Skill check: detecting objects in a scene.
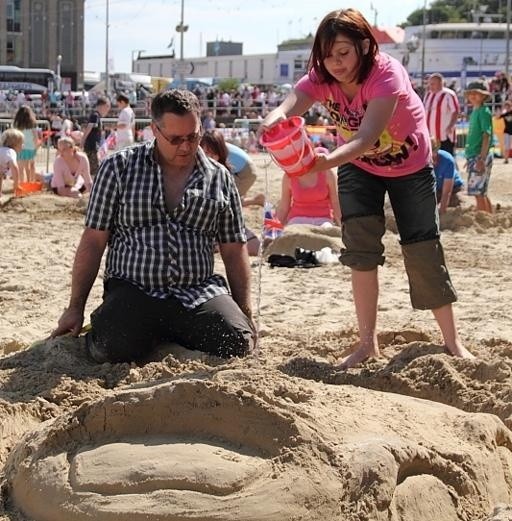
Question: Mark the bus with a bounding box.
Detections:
[0,66,61,93]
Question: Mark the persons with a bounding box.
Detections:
[53,88,256,363]
[258,8,478,370]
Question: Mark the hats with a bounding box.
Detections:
[461,79,493,103]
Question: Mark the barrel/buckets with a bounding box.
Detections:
[259,115,318,177]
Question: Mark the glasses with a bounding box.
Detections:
[151,119,205,147]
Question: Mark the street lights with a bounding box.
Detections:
[367,3,378,25]
[420,0,429,90]
[57,54,63,92]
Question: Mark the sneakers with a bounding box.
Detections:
[83,331,110,366]
[266,253,296,268]
[295,246,325,266]
